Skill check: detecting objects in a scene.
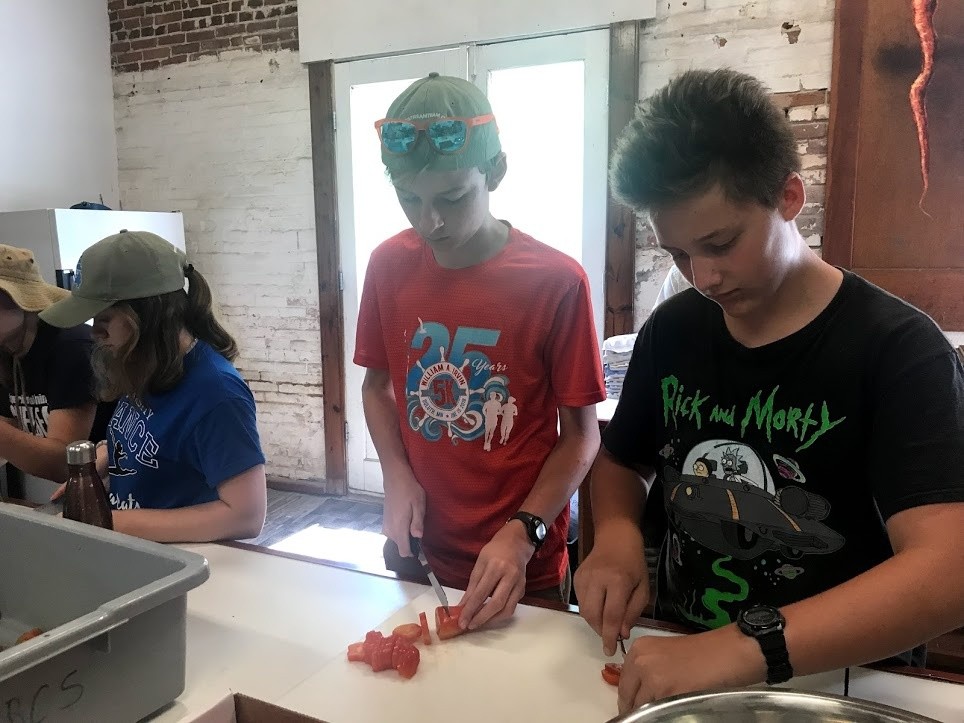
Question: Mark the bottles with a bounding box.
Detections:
[62,440,114,532]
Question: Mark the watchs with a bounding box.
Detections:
[506,511,548,551]
[737,603,793,685]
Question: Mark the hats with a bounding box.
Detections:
[38,229,187,328]
[382,72,502,171]
[0,243,71,312]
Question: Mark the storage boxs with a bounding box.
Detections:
[0,500,209,722]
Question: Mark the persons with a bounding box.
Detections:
[352,73,607,632]
[49,231,267,543]
[570,66,964,717]
[0,244,121,485]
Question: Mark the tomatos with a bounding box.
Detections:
[347,604,486,679]
[601,661,624,685]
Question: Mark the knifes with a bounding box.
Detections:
[409,533,448,613]
[617,634,628,660]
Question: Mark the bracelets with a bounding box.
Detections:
[96,440,107,449]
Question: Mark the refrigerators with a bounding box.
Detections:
[0,207,190,326]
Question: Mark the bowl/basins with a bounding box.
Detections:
[605,687,945,723]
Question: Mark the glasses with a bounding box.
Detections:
[374,114,497,156]
